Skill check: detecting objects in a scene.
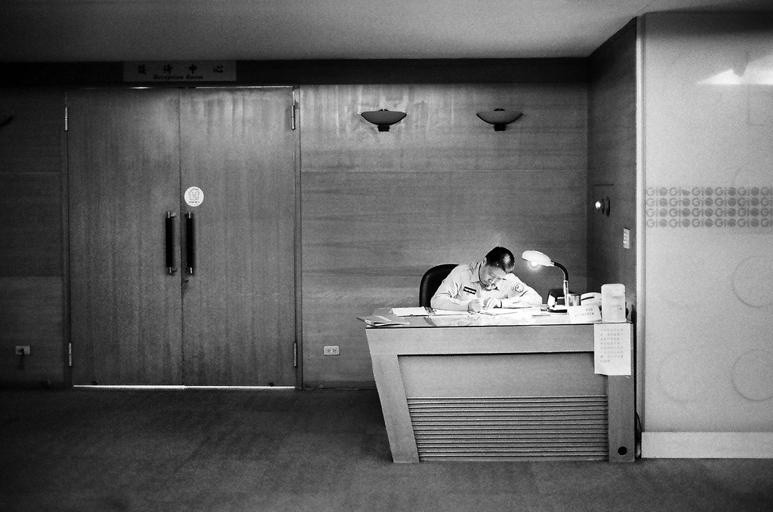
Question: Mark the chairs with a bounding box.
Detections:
[419,264,459,306]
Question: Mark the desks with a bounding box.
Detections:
[365,306,635,463]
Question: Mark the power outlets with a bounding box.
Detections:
[323,346,339,356]
[15,345,31,356]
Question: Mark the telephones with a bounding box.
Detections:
[580,292,603,307]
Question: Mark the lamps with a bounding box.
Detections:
[592,198,612,215]
[361,109,406,132]
[477,108,522,131]
[521,250,571,317]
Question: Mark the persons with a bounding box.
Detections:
[430,246,543,312]
